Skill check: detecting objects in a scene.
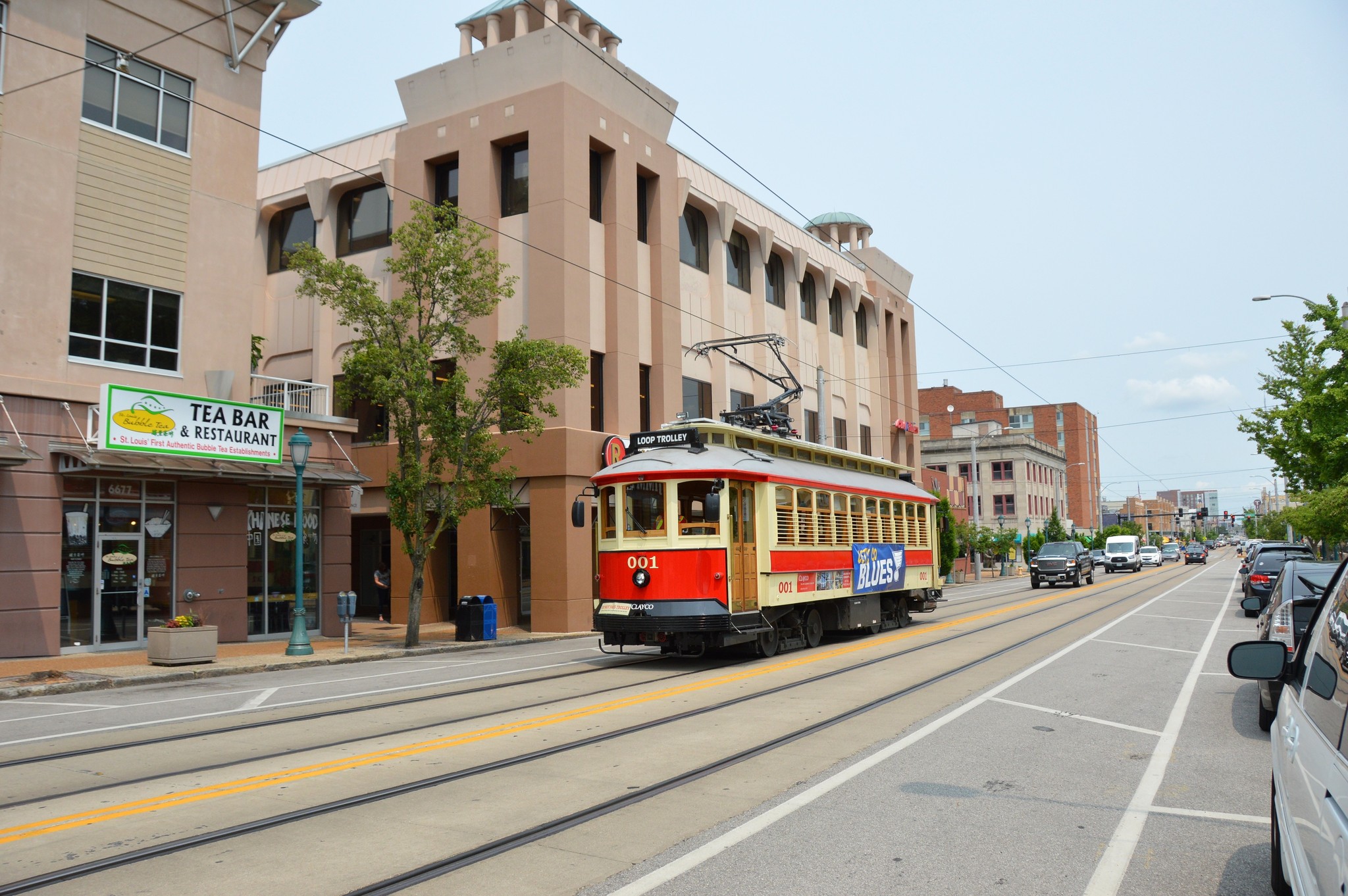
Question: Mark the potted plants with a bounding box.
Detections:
[1005,564,1015,576]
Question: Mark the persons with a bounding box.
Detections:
[374,562,390,621]
[653,494,687,529]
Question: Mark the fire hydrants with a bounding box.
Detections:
[1017,564,1024,575]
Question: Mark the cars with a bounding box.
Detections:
[1238,560,1344,729]
[1226,556,1348,896]
[1327,608,1348,666]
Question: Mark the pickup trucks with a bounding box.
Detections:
[1029,534,1319,614]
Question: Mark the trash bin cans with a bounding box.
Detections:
[455,596,484,641]
[477,595,496,640]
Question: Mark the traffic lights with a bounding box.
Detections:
[1173,531,1184,538]
[1197,512,1203,520]
[1224,511,1251,528]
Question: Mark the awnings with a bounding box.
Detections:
[0,444,44,465]
[50,446,374,487]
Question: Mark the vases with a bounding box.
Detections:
[148,626,218,663]
[951,572,964,583]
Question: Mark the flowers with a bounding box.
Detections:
[955,569,963,573]
[161,611,205,628]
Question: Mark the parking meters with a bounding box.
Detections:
[337,591,356,653]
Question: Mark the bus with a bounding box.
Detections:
[571,334,949,660]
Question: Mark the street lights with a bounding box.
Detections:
[1099,482,1122,533]
[1145,501,1162,546]
[1250,475,1279,515]
[1025,517,1032,572]
[997,514,1007,576]
[1089,525,1094,551]
[1043,519,1050,544]
[1127,493,1147,522]
[1250,452,1293,543]
[972,427,1014,578]
[1249,496,1265,514]
[1160,505,1175,546]
[283,425,315,655]
[1072,524,1076,541]
[1249,488,1273,518]
[1056,462,1086,525]
